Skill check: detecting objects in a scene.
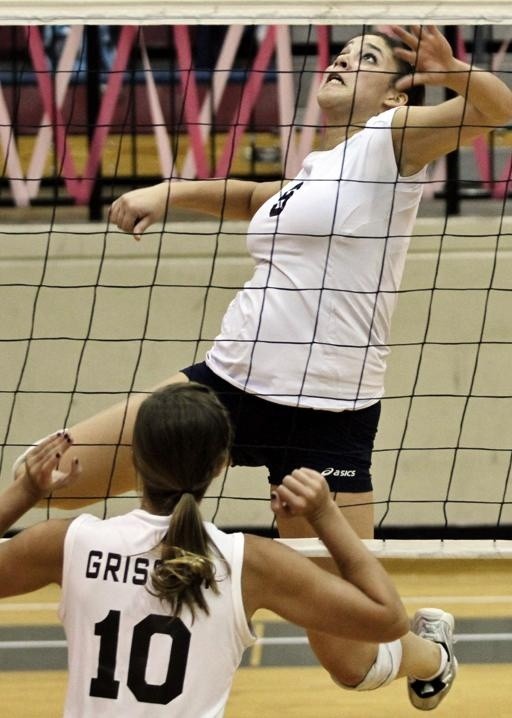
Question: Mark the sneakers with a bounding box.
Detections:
[409,609,455,712]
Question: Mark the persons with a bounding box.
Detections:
[2,379,414,717]
[14,22,509,712]
